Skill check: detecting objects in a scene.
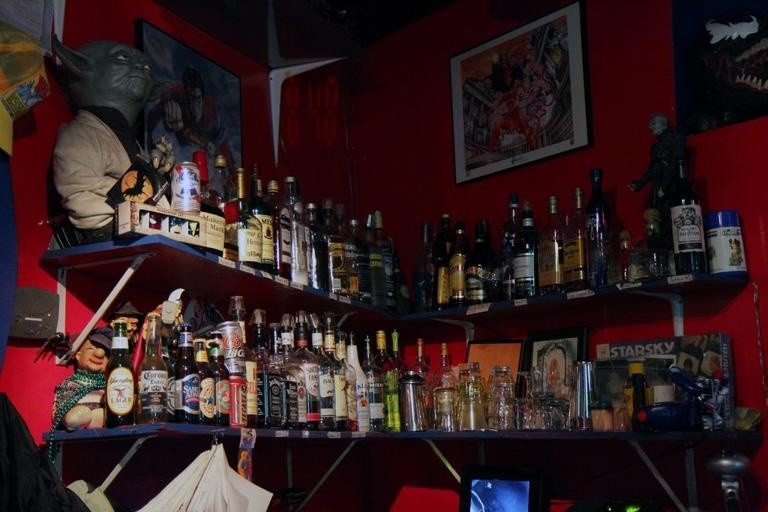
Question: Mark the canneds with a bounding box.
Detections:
[170,161,203,214]
[228,377,249,427]
[215,320,247,375]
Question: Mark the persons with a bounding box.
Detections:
[674,208,702,228]
[52,286,186,430]
[626,117,691,229]
[148,66,239,172]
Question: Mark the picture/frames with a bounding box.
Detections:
[447,1,594,185]
[514,327,589,428]
[134,17,244,188]
[464,337,526,399]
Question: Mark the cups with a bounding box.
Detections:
[149,210,161,229]
[169,215,182,234]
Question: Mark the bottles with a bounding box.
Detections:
[137,179,170,217]
[191,144,407,309]
[666,158,709,276]
[410,164,665,314]
[103,293,657,430]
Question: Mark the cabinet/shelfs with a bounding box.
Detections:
[39,233,397,443]
[396,270,760,443]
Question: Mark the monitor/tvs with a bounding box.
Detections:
[459,463,545,512]
